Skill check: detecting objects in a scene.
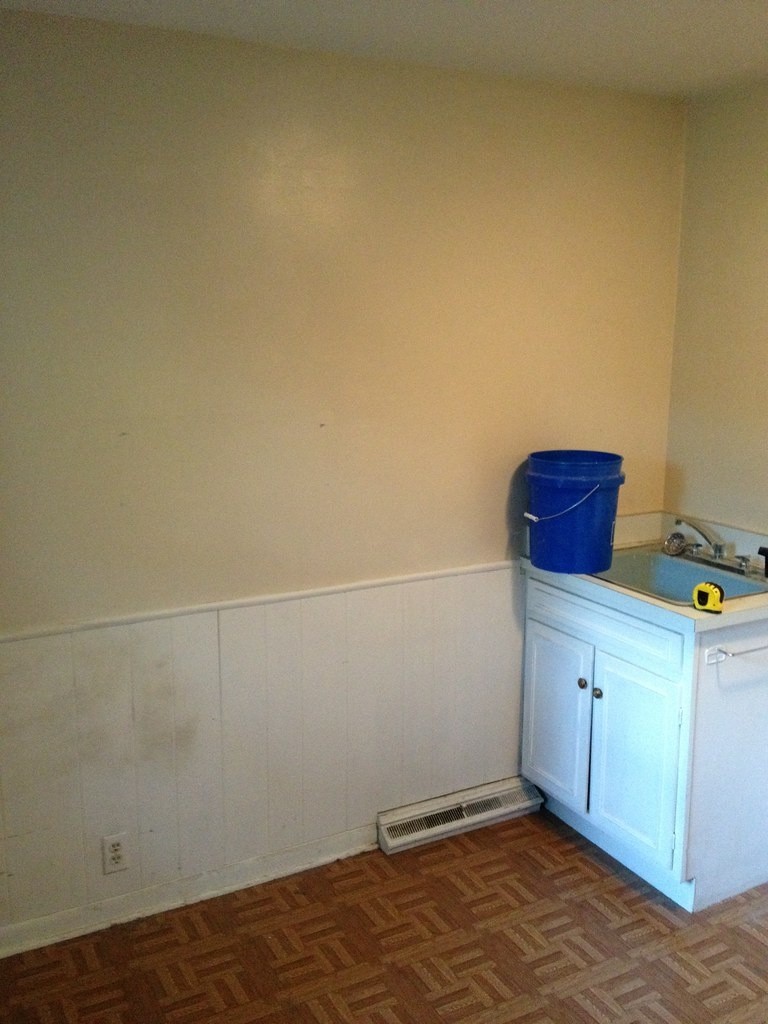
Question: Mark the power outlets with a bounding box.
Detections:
[101,832,129,875]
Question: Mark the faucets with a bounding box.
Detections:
[673,518,726,557]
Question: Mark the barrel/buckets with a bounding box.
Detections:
[524,451,625,574]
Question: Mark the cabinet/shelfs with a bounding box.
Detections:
[521,577,768,913]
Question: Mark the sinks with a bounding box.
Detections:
[581,540,768,605]
